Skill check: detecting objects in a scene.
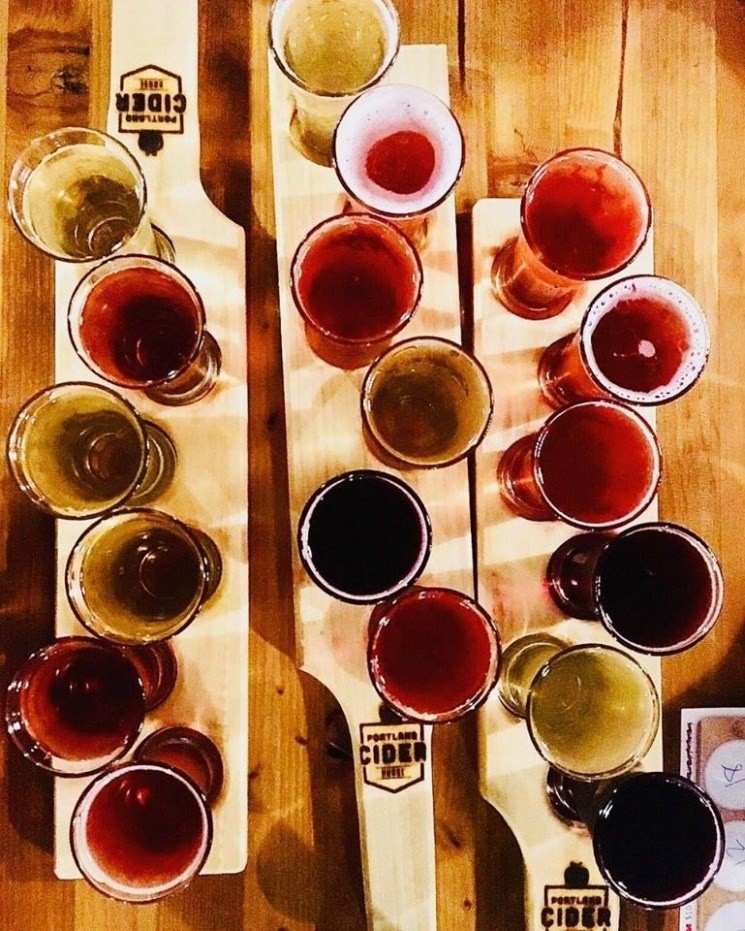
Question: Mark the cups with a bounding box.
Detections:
[296,469,432,605]
[65,508,210,646]
[289,212,425,370]
[267,0,400,153]
[509,398,664,532]
[66,253,216,400]
[365,586,503,725]
[360,336,494,471]
[3,381,153,520]
[545,522,725,656]
[5,635,160,777]
[68,744,214,905]
[331,83,466,236]
[496,632,660,782]
[7,126,159,273]
[512,147,652,307]
[546,762,726,910]
[544,274,711,405]
